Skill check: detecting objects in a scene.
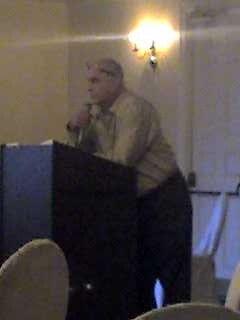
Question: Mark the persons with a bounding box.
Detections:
[61,59,192,313]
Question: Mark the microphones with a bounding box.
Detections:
[76,102,92,146]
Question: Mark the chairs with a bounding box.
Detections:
[1,239,71,319]
[135,303,239,319]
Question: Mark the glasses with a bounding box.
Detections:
[85,61,113,77]
